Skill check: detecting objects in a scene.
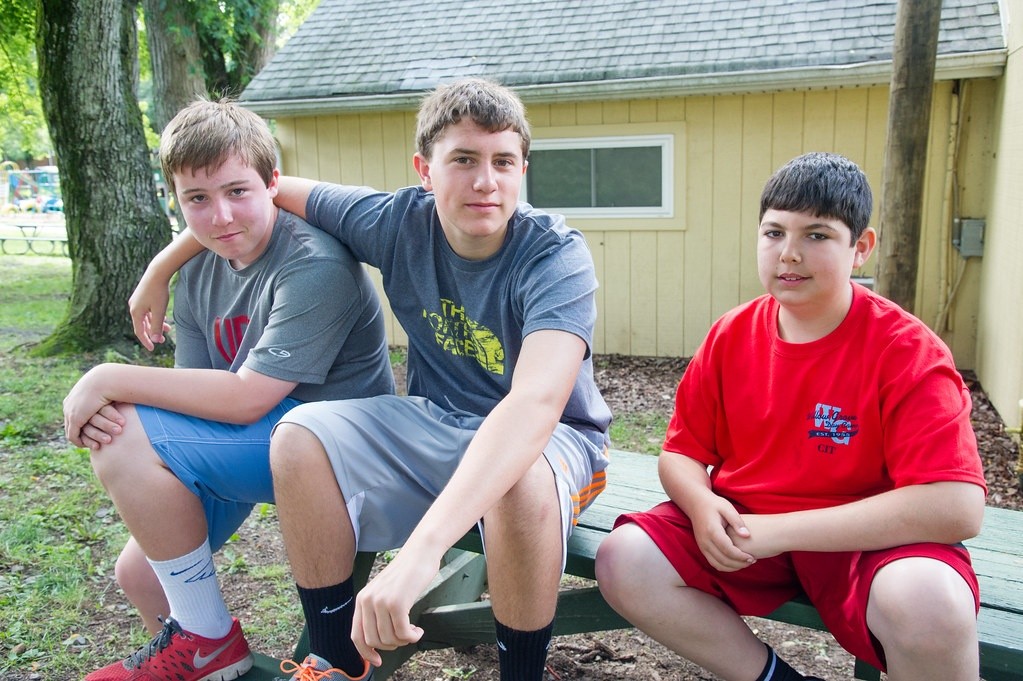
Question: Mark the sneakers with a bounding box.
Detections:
[81,615,253,681]
[279,652,375,681]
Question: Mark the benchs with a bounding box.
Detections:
[238,450,1022,681]
[0,238,69,257]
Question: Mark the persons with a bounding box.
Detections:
[129,78,613,681]
[594,153,988,680]
[63,103,396,680]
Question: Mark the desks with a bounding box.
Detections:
[17,225,39,255]
